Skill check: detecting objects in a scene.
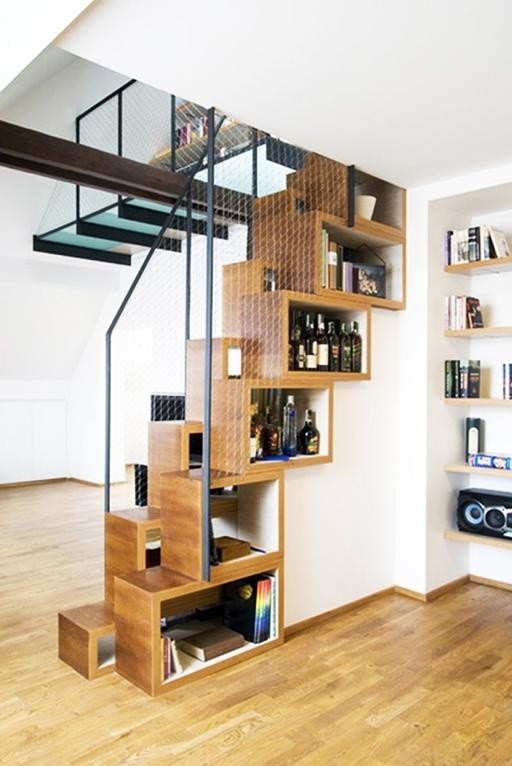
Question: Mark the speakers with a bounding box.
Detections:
[455,483,512,540]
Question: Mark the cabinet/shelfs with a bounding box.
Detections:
[113,164,407,697]
[441,254,509,548]
[58,153,318,682]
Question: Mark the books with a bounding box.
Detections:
[446,225,509,265]
[447,294,485,329]
[177,628,245,662]
[446,359,482,398]
[162,635,183,681]
[219,574,275,642]
[322,226,352,292]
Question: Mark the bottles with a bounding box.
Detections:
[316,314,329,371]
[338,323,352,372]
[299,409,319,455]
[282,395,297,457]
[254,402,260,461]
[348,321,362,372]
[291,309,305,371]
[303,314,319,371]
[260,406,279,457]
[326,322,338,371]
[250,405,256,463]
